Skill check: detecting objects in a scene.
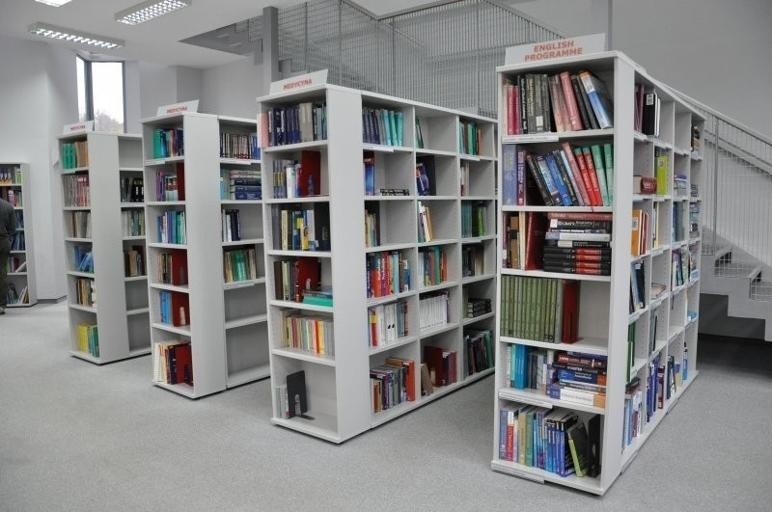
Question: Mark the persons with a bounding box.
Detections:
[0,197,19,316]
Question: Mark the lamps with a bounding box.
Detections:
[35,0,74,10]
[117,0,192,27]
[27,24,126,53]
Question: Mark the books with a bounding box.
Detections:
[60,140,101,359]
[621,76,702,457]
[0,164,30,305]
[219,126,263,284]
[457,121,495,380]
[502,143,614,207]
[150,124,195,387]
[256,99,336,421]
[416,116,459,395]
[503,71,612,135]
[119,172,148,278]
[502,212,613,478]
[360,104,416,416]
[623,76,650,455]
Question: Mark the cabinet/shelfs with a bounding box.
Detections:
[253,82,497,446]
[139,111,269,400]
[494,51,708,494]
[2,162,38,308]
[56,130,152,365]
